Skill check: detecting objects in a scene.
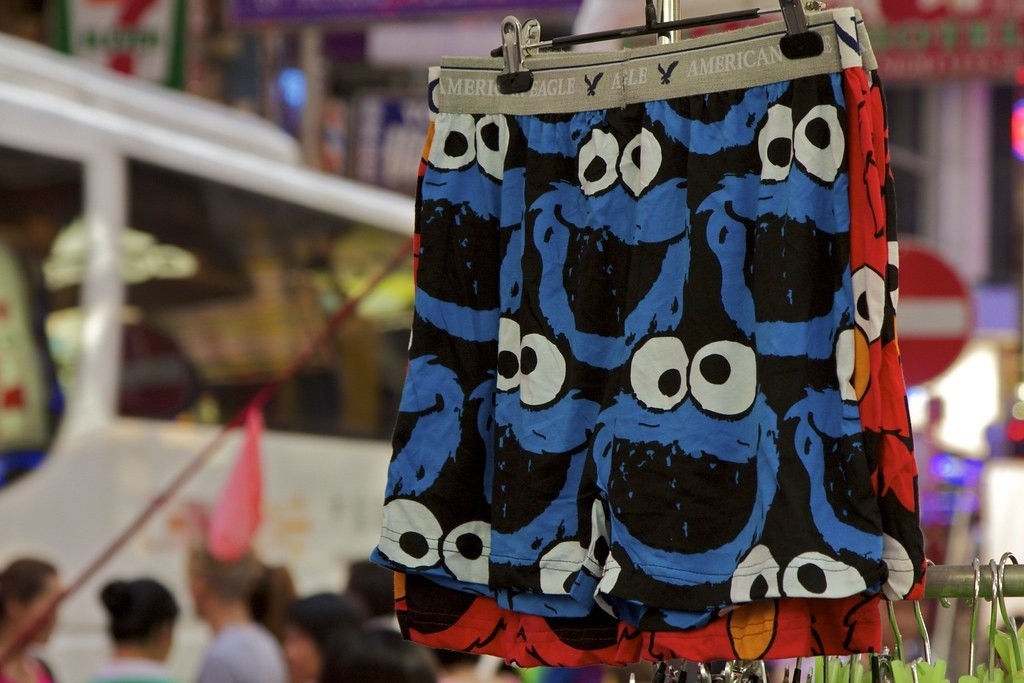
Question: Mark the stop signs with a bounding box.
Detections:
[893,246,979,394]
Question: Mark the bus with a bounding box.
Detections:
[0,35,427,672]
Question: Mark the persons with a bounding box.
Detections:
[185,536,482,683]
[87,579,178,683]
[911,396,945,564]
[0,559,62,683]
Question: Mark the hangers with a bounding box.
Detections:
[488,0,826,94]
[647,554,1024,683]
